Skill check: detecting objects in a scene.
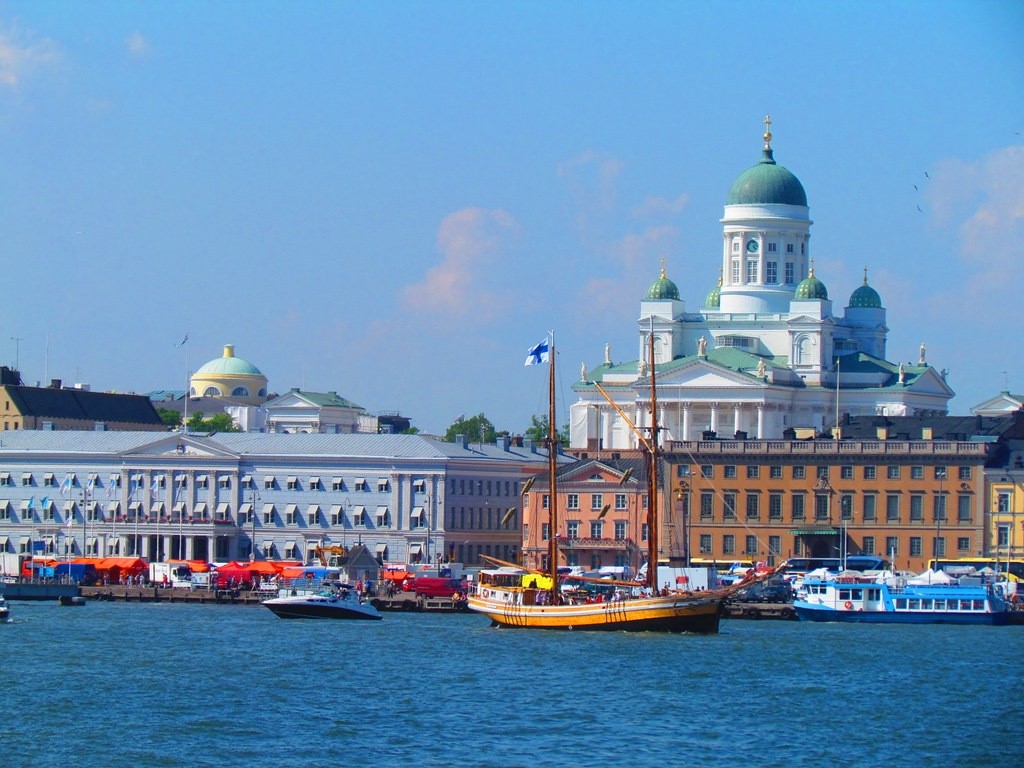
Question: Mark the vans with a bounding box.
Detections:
[415,577,465,600]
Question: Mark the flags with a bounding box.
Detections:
[128,479,137,500]
[175,483,181,503]
[524,337,549,366]
[66,516,72,527]
[28,497,34,508]
[41,496,48,509]
[106,478,115,497]
[86,476,93,492]
[60,473,71,494]
[149,481,158,500]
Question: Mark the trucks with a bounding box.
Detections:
[152,565,193,587]
[1,553,39,584]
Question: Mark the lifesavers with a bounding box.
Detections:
[403,599,413,610]
[845,601,852,609]
[370,598,381,609]
[781,607,792,618]
[720,608,727,618]
[483,589,489,598]
[747,608,759,619]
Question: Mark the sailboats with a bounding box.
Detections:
[465,315,792,634]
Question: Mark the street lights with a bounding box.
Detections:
[79,486,430,564]
[684,471,696,568]
[936,470,945,558]
[993,495,1006,567]
[838,491,847,571]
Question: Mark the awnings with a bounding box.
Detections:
[0,472,424,554]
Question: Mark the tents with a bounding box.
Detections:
[22,558,302,588]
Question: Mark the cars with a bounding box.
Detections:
[0,596,11,619]
[561,572,617,595]
[739,583,789,603]
[402,579,415,592]
[460,580,475,588]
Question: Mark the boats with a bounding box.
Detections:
[260,578,384,623]
[794,568,1012,625]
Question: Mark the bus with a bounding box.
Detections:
[639,557,756,576]
[315,547,349,568]
[783,557,892,572]
[928,558,1024,590]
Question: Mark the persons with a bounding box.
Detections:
[899,363,904,382]
[162,573,167,588]
[920,343,925,362]
[536,585,667,606]
[251,576,258,591]
[581,335,766,380]
[356,579,468,601]
[941,369,948,379]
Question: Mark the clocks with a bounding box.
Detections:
[746,239,760,252]
